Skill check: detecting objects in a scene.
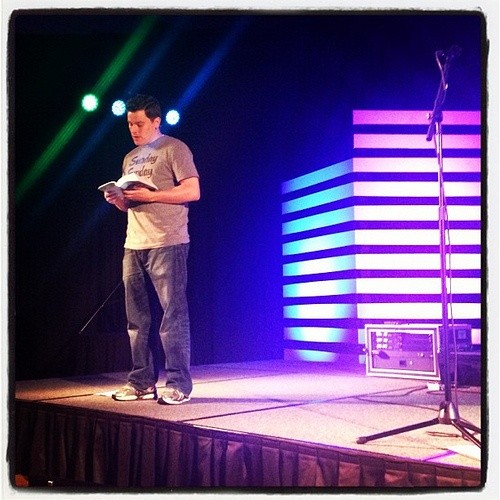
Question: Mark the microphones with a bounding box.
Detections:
[436,49,451,90]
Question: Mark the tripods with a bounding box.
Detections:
[357,82,483,452]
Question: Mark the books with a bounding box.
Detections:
[99,173,160,195]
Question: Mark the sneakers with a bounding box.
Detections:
[158,387,191,404]
[112,382,157,402]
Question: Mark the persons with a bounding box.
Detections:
[103,93,203,405]
